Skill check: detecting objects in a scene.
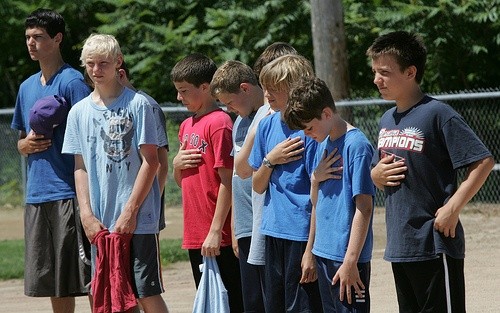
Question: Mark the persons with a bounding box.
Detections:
[367,29,496,312]
[171,40,373,312]
[11,6,170,312]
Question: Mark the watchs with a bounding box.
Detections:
[263,157,274,168]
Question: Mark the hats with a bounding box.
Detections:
[29,95,68,140]
